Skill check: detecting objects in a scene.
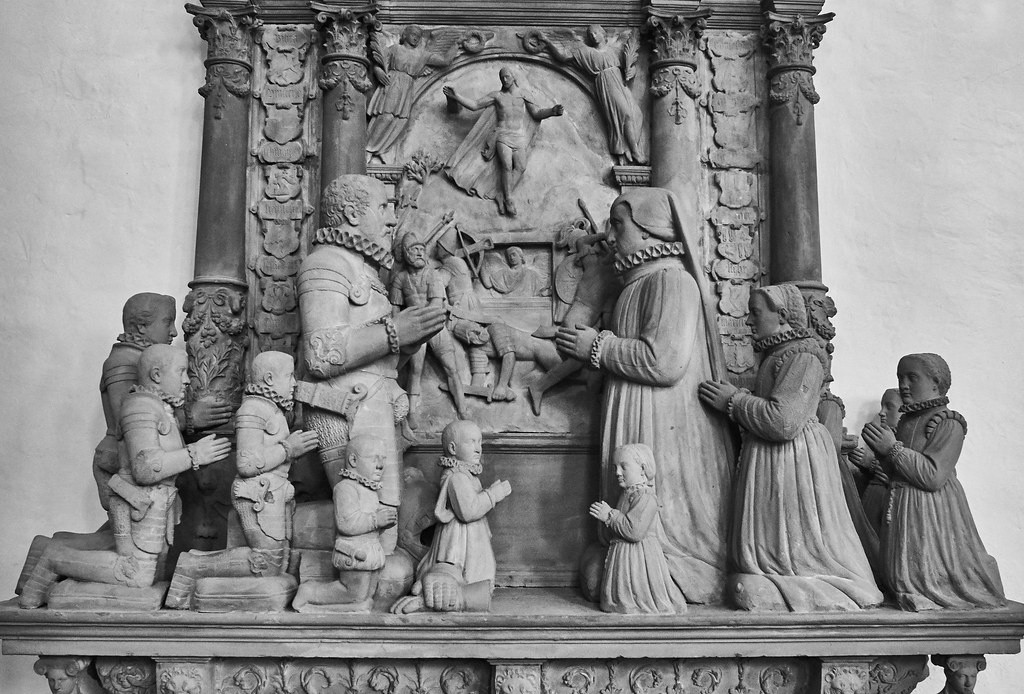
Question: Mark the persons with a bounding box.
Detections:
[529,187,1007,613]
[443,66,564,216]
[540,25,649,166]
[17,173,512,613]
[480,247,552,299]
[454,320,602,400]
[364,25,467,165]
